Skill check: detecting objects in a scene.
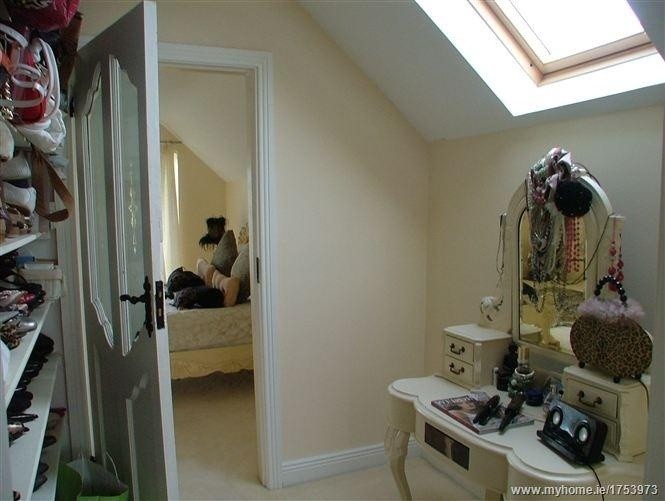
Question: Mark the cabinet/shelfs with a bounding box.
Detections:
[561,362,653,464]
[0,105,70,501]
[440,324,512,393]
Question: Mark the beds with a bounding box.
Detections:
[162,220,254,384]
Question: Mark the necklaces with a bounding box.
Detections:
[573,216,580,272]
[608,216,625,292]
[533,210,574,314]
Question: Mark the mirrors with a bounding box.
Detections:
[500,164,626,398]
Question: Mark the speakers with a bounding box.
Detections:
[536,398,609,466]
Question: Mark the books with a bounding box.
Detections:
[431,390,534,435]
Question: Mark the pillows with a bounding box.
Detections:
[197,220,252,307]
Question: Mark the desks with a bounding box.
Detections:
[378,373,649,501]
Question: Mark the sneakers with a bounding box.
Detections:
[1,152,37,243]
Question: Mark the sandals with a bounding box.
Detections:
[33,408,60,492]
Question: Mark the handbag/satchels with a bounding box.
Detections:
[1,0,83,153]
[570,275,653,382]
[56,448,130,501]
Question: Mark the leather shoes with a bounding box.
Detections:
[1,269,46,349]
[6,334,54,440]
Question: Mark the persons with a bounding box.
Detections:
[449,400,484,425]
